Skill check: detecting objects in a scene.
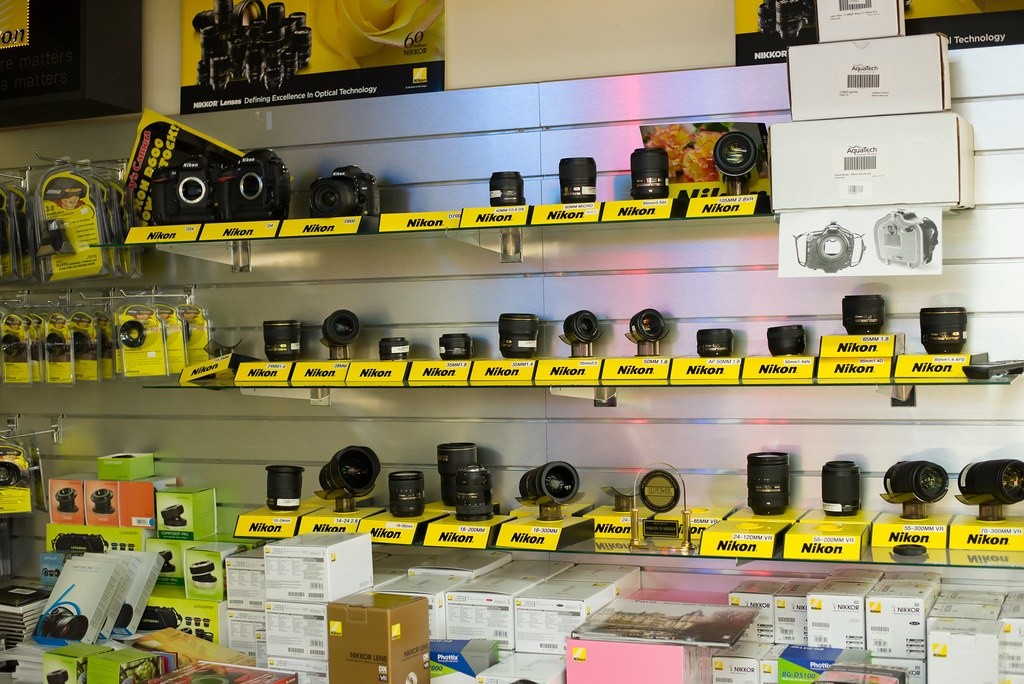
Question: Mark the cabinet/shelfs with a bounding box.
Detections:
[89,213,1024,569]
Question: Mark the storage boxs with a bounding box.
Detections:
[41,452,1024,684]
[0,486,33,513]
[768,0,976,209]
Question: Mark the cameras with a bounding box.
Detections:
[151,156,222,225]
[308,166,380,218]
[875,211,939,265]
[35,608,88,640]
[212,149,291,221]
[804,224,855,273]
